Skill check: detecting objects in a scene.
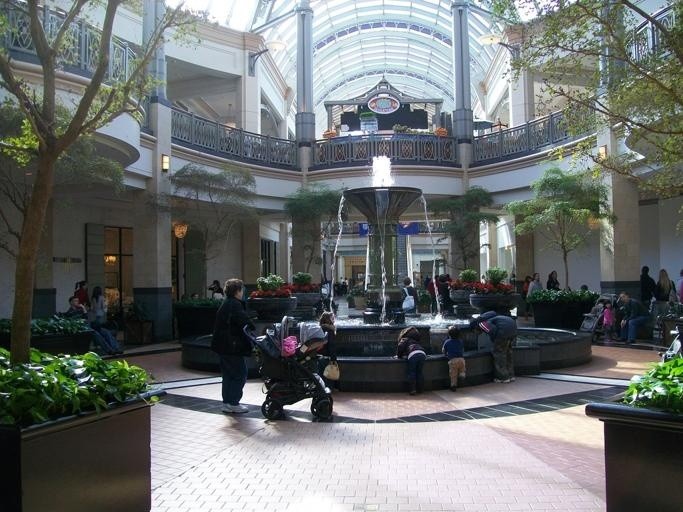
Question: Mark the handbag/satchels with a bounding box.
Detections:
[227,300,249,354]
[521,291,526,300]
[402,288,414,310]
[323,360,340,381]
[668,280,679,307]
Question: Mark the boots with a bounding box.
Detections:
[525,312,528,321]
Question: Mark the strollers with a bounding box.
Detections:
[579,292,621,342]
[659,315,682,363]
[242,315,336,420]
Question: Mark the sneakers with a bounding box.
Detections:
[493,377,510,383]
[450,387,456,391]
[510,376,515,382]
[332,388,339,392]
[224,403,248,414]
[221,402,226,411]
[603,338,635,343]
[460,380,465,384]
[410,389,416,395]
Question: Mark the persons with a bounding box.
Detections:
[192,278,248,309]
[395,326,426,394]
[442,326,466,391]
[317,311,340,393]
[339,276,357,295]
[402,272,454,314]
[522,269,589,321]
[212,279,254,413]
[469,310,517,383]
[67,280,123,356]
[603,265,683,345]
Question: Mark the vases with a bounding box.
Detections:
[291,292,320,309]
[466,292,522,316]
[448,289,477,305]
[247,296,298,320]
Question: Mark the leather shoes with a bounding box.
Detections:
[115,350,123,353]
[108,350,115,355]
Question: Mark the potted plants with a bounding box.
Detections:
[0,1,216,511]
[490,0,683,512]
[501,165,618,333]
[415,289,432,314]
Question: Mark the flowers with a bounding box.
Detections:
[473,266,511,294]
[451,268,478,290]
[244,274,297,298]
[290,270,321,293]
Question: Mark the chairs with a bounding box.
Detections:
[642,295,670,347]
